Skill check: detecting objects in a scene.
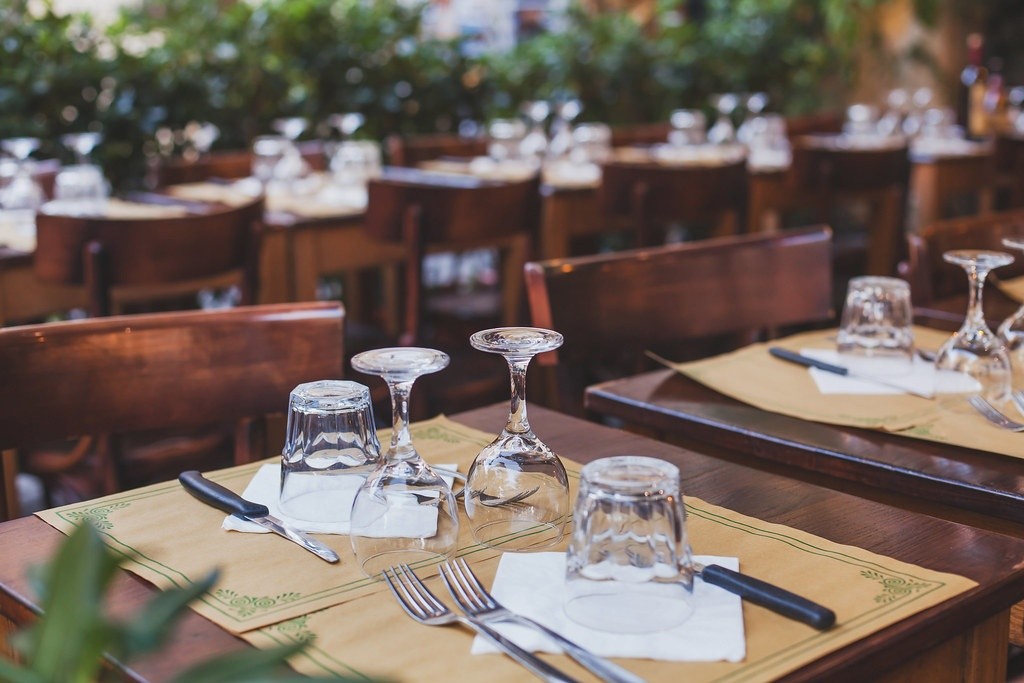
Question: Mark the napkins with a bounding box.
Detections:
[797,343,985,399]
[466,549,747,664]
[219,461,461,540]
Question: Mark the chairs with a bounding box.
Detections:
[905,212,1024,304]
[362,150,546,350]
[34,182,271,317]
[520,221,836,412]
[0,159,63,201]
[583,143,751,255]
[979,132,1024,209]
[787,134,913,282]
[0,300,347,521]
[610,129,671,147]
[139,145,329,194]
[786,108,849,136]
[382,133,487,169]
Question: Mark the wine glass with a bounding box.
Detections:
[350,346,459,582]
[1,132,107,238]
[664,89,789,163]
[997,236,1024,370]
[934,250,1015,416]
[465,327,570,551]
[488,98,611,178]
[248,114,382,206]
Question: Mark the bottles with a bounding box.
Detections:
[966,32,1006,136]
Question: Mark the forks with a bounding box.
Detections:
[970,394,1024,432]
[382,563,580,683]
[439,558,645,683]
[426,463,540,507]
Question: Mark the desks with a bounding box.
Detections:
[0,131,1024,683]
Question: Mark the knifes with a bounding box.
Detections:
[770,346,932,400]
[179,470,339,563]
[691,559,836,631]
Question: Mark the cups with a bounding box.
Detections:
[836,275,913,356]
[277,380,381,522]
[562,456,702,633]
